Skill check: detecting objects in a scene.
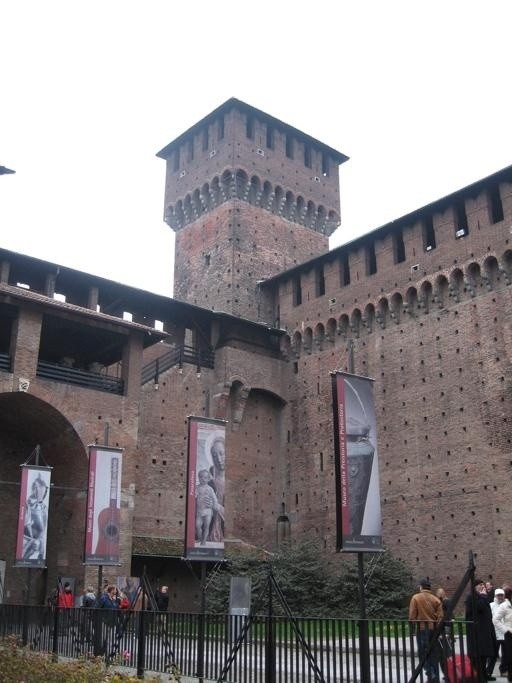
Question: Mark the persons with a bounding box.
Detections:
[201,431,225,542]
[434,588,454,683]
[39,574,176,657]
[464,579,511,683]
[195,468,217,545]
[409,576,444,683]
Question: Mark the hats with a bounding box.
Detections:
[474,579,483,586]
[495,589,504,595]
[418,578,431,586]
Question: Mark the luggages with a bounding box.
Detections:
[439,636,478,683]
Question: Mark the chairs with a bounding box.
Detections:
[48,354,75,383]
[79,360,105,389]
